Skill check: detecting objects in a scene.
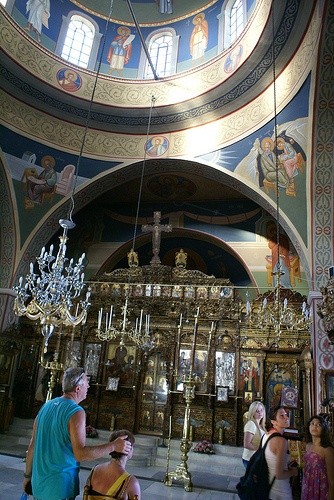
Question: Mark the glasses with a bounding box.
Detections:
[73,367,85,386]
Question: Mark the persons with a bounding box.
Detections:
[300,415,334,500]
[242,401,268,467]
[22,366,132,499]
[82,430,142,500]
[260,406,298,500]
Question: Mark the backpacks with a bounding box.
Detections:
[234,432,291,500]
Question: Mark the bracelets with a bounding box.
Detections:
[24,472,31,478]
